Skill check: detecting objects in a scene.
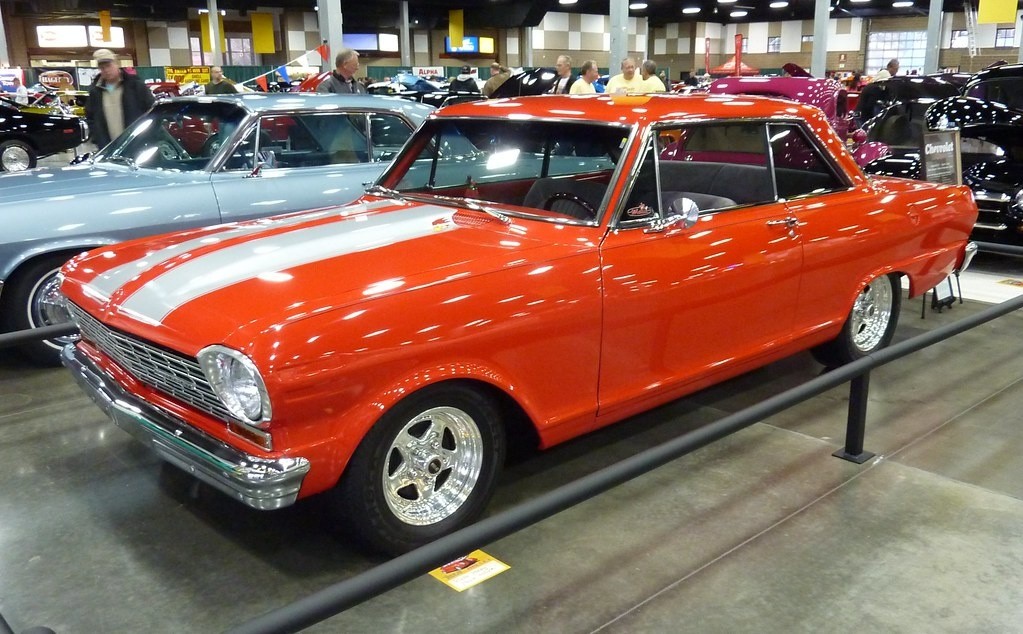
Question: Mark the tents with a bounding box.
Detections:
[708,55,760,75]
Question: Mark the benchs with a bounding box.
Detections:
[639,161,842,203]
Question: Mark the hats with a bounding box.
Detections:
[462,65,470,73]
[94,48,116,64]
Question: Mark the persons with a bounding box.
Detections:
[12,78,29,104]
[569,60,600,94]
[316,47,368,135]
[603,56,666,93]
[543,54,576,95]
[873,59,900,82]
[483,62,512,97]
[448,64,481,94]
[83,49,155,151]
[205,66,247,158]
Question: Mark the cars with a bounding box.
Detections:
[0,91,615,368]
[53,92,982,562]
[0,59,1022,266]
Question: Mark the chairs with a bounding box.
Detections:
[521,177,608,221]
[642,191,737,217]
